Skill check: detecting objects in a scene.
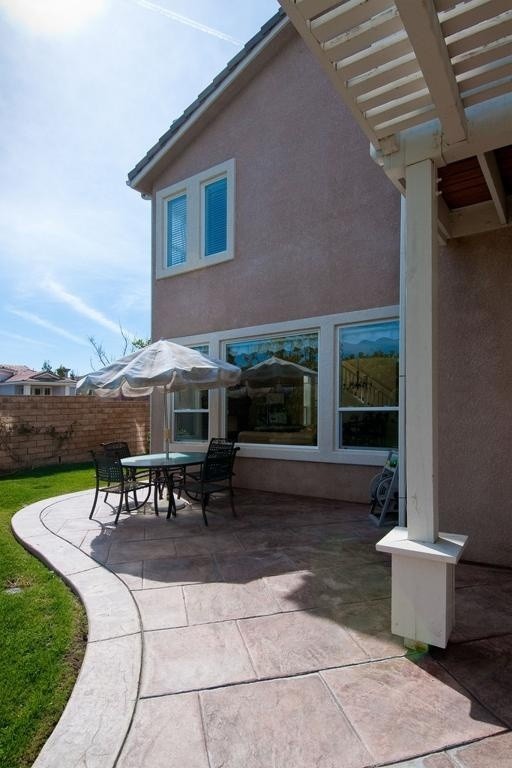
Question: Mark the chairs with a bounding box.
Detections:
[86,437,241,527]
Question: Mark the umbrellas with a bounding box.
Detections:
[73,335,242,461]
[229,353,318,381]
